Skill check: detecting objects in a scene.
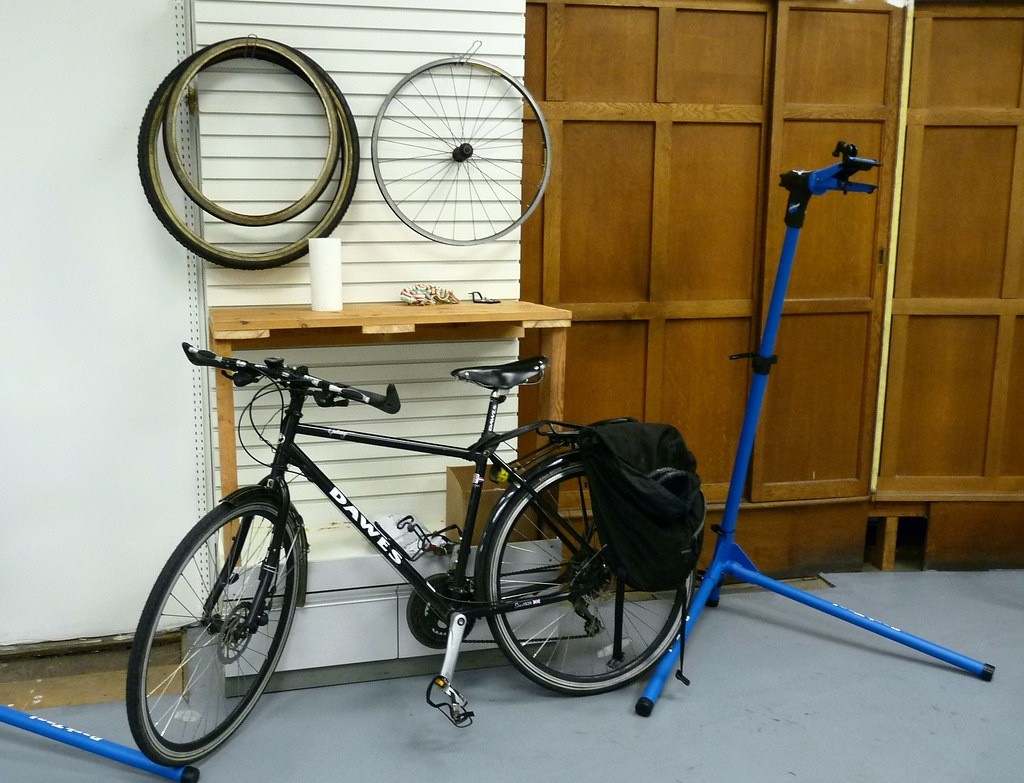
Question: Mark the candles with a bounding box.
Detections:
[308,237,343,312]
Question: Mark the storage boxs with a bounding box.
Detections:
[446,465,537,548]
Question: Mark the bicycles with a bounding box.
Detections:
[124,341,698,768]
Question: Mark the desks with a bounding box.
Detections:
[208,299,572,698]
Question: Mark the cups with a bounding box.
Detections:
[308,236,342,311]
